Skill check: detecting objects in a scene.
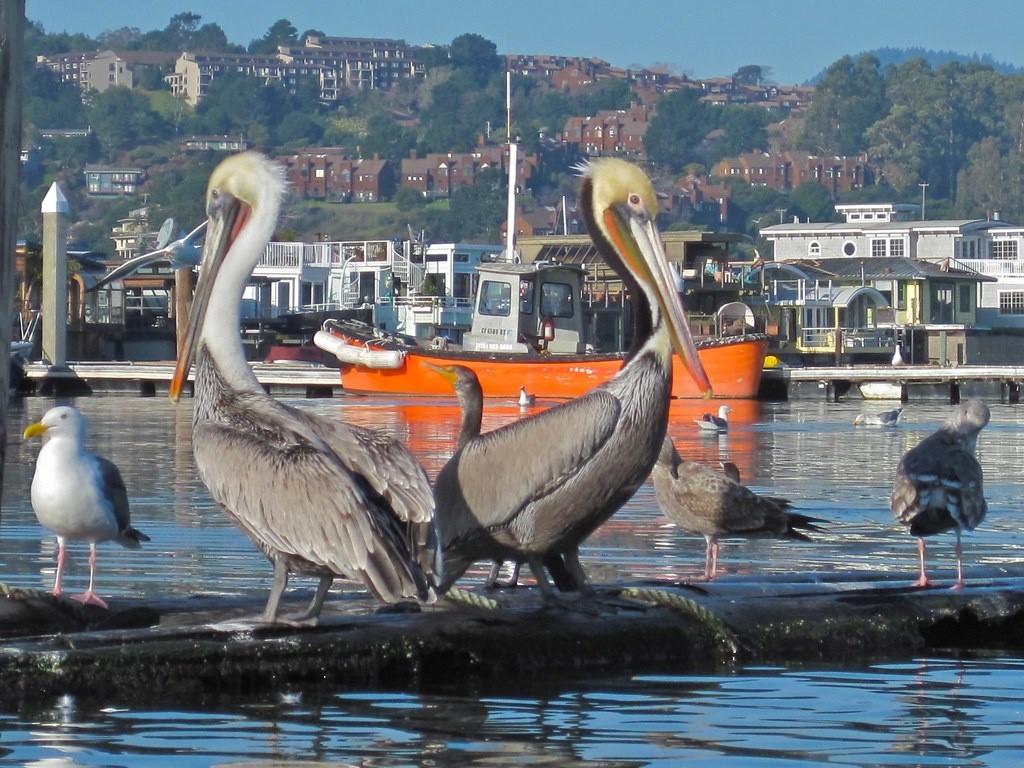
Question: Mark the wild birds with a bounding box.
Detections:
[851,405,905,426]
[23,406,152,609]
[887,399,994,593]
[891,345,903,371]
[175,149,439,638]
[422,152,835,619]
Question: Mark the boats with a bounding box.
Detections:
[8,312,40,360]
[314,143,771,399]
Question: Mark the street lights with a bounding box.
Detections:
[918,181,929,220]
